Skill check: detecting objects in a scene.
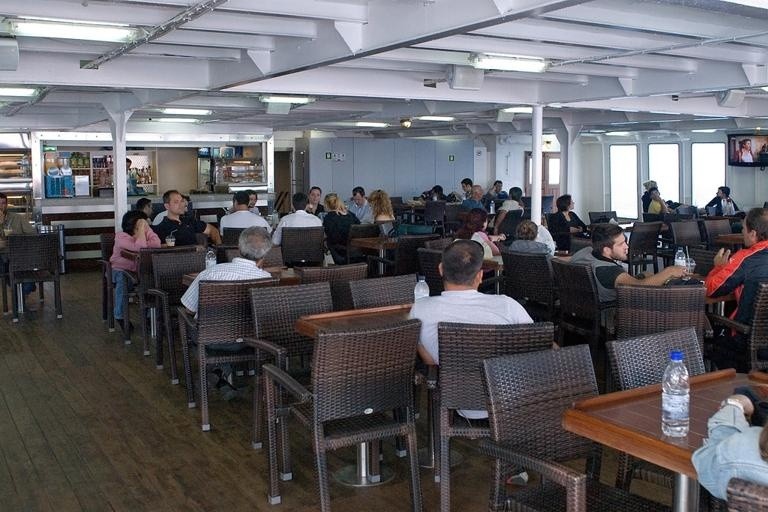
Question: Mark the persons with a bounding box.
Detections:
[462,178,473,199]
[457,208,501,280]
[157,190,221,247]
[368,191,395,222]
[462,184,484,209]
[641,182,673,213]
[109,210,161,333]
[408,240,533,485]
[0,193,40,313]
[485,180,508,210]
[705,187,739,217]
[152,195,188,226]
[227,190,259,215]
[568,223,688,332]
[272,192,322,248]
[549,195,592,251]
[733,139,754,162]
[489,187,524,234]
[136,198,153,224]
[690,394,766,502]
[323,193,368,264]
[127,158,132,196]
[762,144,766,153]
[705,208,767,360]
[219,191,272,237]
[304,186,325,217]
[179,226,273,385]
[509,219,551,254]
[418,185,446,221]
[347,186,375,224]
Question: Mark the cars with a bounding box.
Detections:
[165,235,176,247]
[344,202,348,209]
[681,259,695,281]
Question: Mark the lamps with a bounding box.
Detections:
[0,231,63,322]
[3,18,149,45]
[258,95,314,105]
[467,51,551,75]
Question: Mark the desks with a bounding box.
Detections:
[0,236,40,322]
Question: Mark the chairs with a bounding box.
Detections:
[0,231,63,322]
[96,194,768,511]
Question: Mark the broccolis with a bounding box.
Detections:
[196,146,235,193]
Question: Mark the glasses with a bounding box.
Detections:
[467,51,551,75]
[258,95,314,105]
[3,18,149,45]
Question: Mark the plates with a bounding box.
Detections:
[66,152,115,197]
[210,157,267,193]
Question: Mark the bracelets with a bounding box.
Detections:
[719,398,744,415]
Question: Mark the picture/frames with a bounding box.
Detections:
[0,236,40,322]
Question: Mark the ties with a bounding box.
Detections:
[727,132,768,167]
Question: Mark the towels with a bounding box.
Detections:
[37,224,65,274]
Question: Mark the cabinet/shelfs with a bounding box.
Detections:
[674,246,685,271]
[204,247,217,271]
[490,200,495,213]
[134,167,152,184]
[660,349,691,435]
[722,199,726,216]
[71,153,105,168]
[414,275,430,301]
[66,152,115,197]
[210,157,267,193]
